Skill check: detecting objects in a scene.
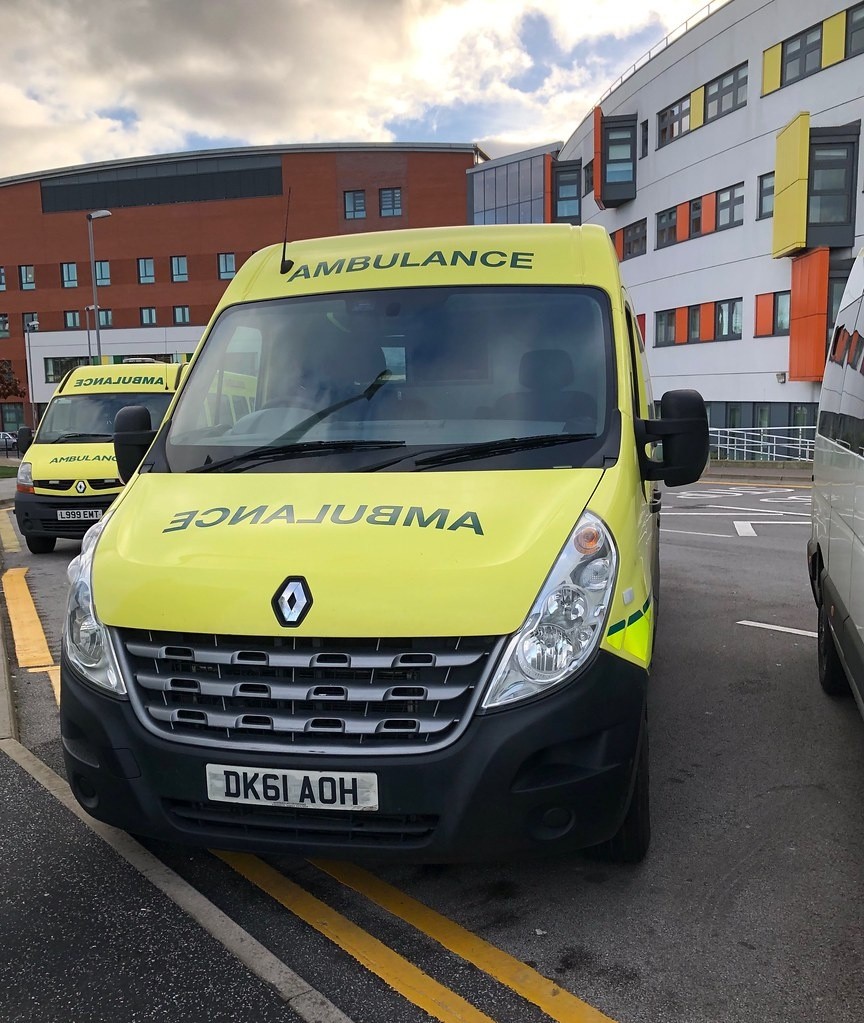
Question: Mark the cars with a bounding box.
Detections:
[0,431,18,451]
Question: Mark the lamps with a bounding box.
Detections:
[776,373,786,384]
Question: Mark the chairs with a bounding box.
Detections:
[495,349,598,422]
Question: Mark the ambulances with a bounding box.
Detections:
[12,357,267,556]
[58,187,708,872]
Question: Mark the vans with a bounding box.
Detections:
[805,245,863,732]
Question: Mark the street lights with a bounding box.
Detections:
[84,304,102,365]
[27,320,40,437]
[85,209,114,362]
